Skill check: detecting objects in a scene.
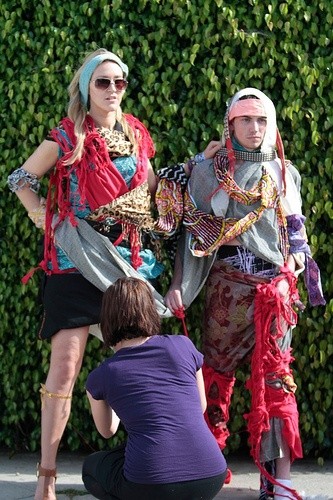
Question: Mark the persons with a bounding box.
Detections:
[82,277,228,500]
[8,50,223,500]
[165,88,326,500]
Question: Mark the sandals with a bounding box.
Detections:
[34,464,58,500]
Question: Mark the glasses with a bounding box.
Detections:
[90,79,128,91]
